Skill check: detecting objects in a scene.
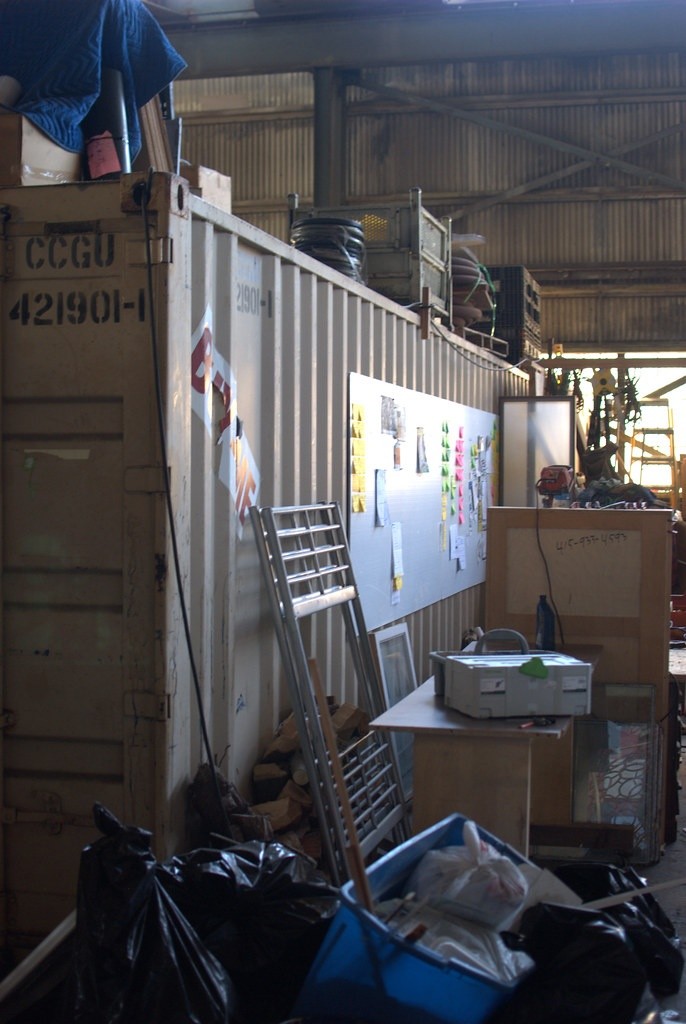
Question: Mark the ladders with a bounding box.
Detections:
[627,396,680,511]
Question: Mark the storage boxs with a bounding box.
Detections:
[289,812,553,1024]
[179,163,232,214]
[0,111,86,187]
[444,650,592,715]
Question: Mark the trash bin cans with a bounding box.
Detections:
[294,812,549,1023]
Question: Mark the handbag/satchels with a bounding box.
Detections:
[402,819,529,931]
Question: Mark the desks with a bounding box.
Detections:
[365,673,574,861]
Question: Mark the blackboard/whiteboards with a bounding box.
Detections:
[347,371,501,637]
